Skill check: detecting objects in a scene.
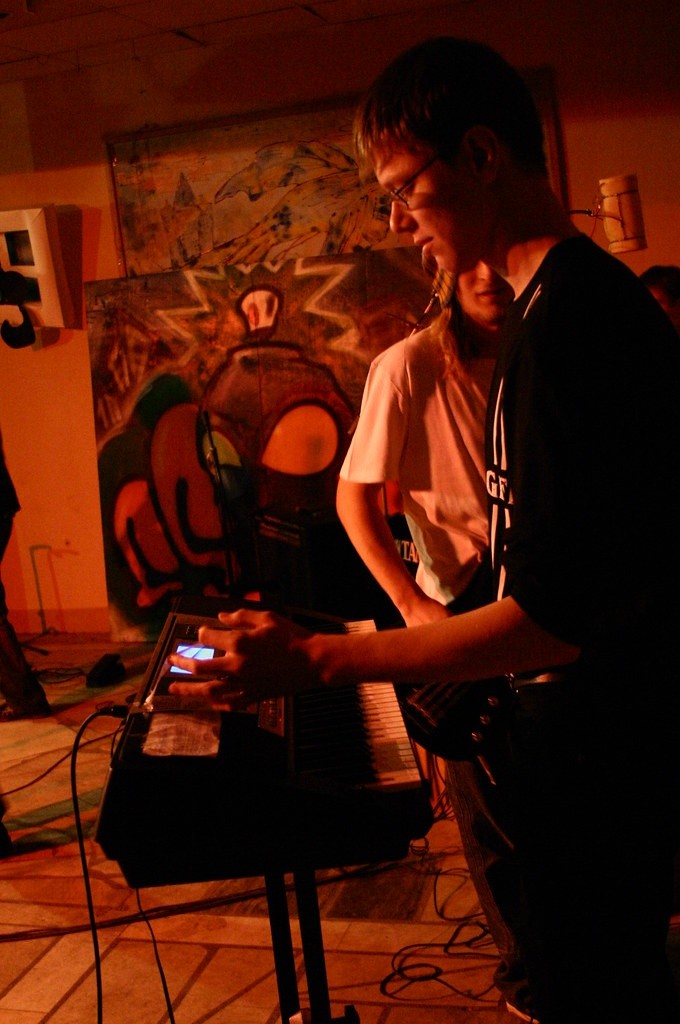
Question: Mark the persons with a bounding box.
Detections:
[335,254,537,1024]
[169,37,680,1024]
[0,423,49,722]
[640,265,680,333]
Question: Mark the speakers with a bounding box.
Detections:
[253,503,400,631]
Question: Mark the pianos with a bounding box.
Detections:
[91,590,437,888]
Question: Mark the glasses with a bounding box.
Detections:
[385,152,440,211]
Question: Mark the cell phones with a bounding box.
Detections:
[167,640,226,681]
